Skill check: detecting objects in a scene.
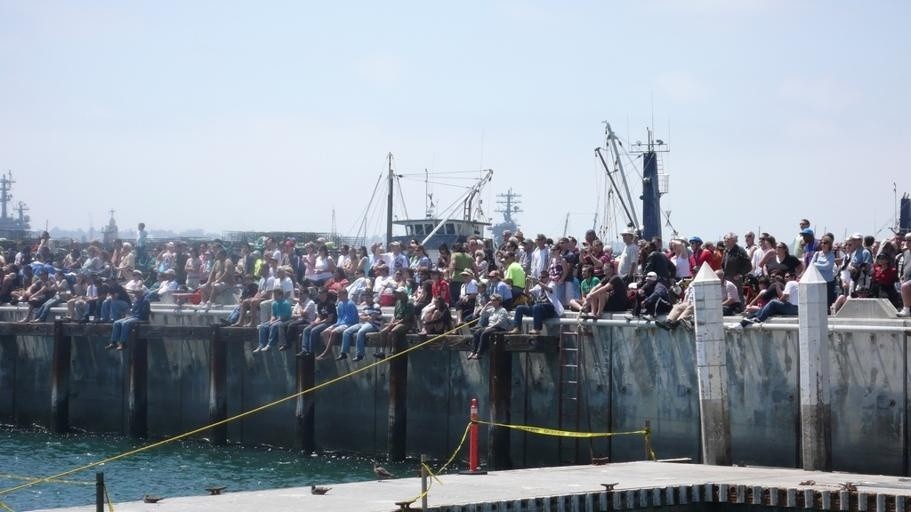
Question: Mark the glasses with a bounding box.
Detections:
[799,222,806,226]
[844,242,853,247]
[744,234,752,238]
[759,237,767,241]
[820,240,828,244]
[768,275,776,278]
[775,246,783,249]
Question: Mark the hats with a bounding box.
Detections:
[486,271,499,277]
[689,237,704,244]
[619,229,637,237]
[876,252,890,260]
[851,233,864,240]
[377,264,390,271]
[164,241,175,248]
[514,231,525,241]
[285,241,294,247]
[459,268,474,277]
[646,272,657,279]
[675,236,691,247]
[316,237,326,243]
[474,250,485,259]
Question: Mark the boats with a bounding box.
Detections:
[391,169,494,248]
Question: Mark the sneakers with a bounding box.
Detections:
[220,317,256,327]
[738,311,761,327]
[579,313,603,319]
[251,344,386,362]
[895,306,911,317]
[190,300,212,310]
[467,352,481,360]
[507,328,541,335]
[623,311,695,334]
[19,315,114,323]
[104,342,126,351]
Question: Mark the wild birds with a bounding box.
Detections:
[372,460,398,481]
[309,483,333,496]
[143,492,168,503]
